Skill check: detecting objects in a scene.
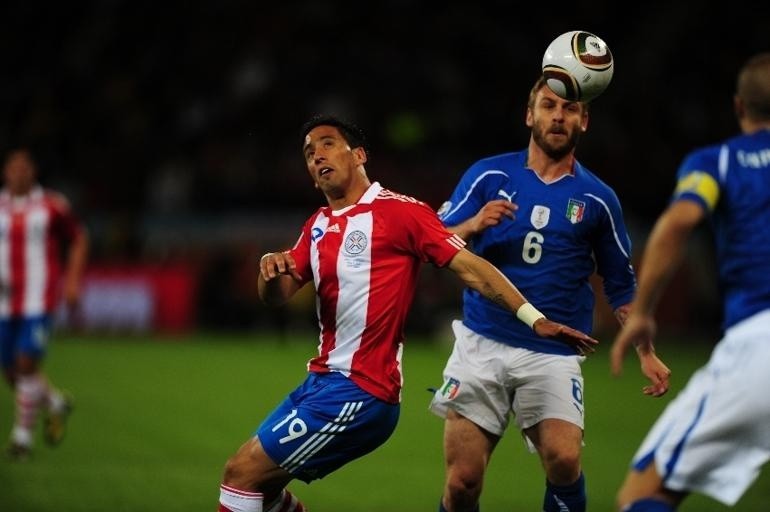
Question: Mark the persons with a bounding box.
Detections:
[438,72,672,510]
[611,53,769,512]
[0,147,89,461]
[217,114,597,511]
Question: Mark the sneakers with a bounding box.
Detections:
[5,394,76,461]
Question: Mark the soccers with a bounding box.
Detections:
[541,28,615,103]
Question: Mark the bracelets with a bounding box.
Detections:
[516,302,545,328]
